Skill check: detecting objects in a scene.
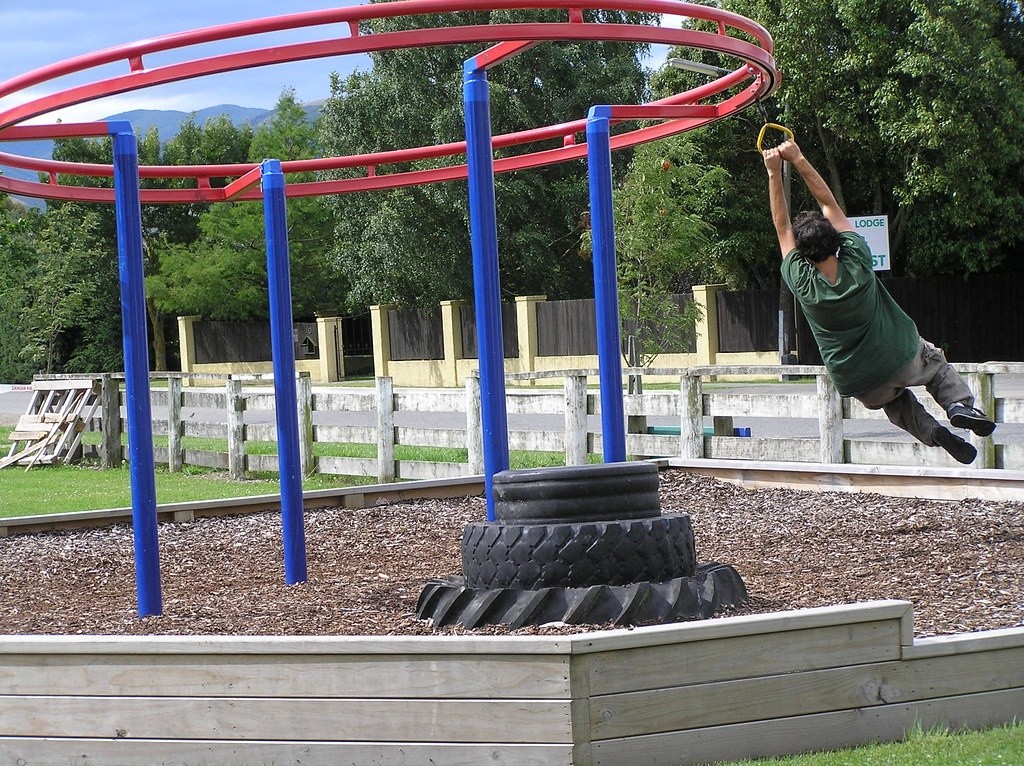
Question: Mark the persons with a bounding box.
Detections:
[762,138,997,465]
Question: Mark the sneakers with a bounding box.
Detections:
[931,426,977,464]
[948,404,996,436]
